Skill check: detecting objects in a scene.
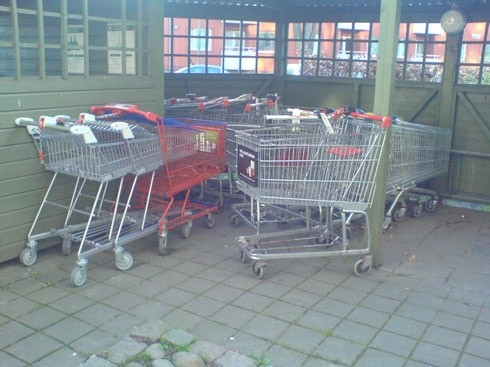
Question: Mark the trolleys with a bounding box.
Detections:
[14,92,451,280]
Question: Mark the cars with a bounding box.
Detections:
[176,64,229,74]
